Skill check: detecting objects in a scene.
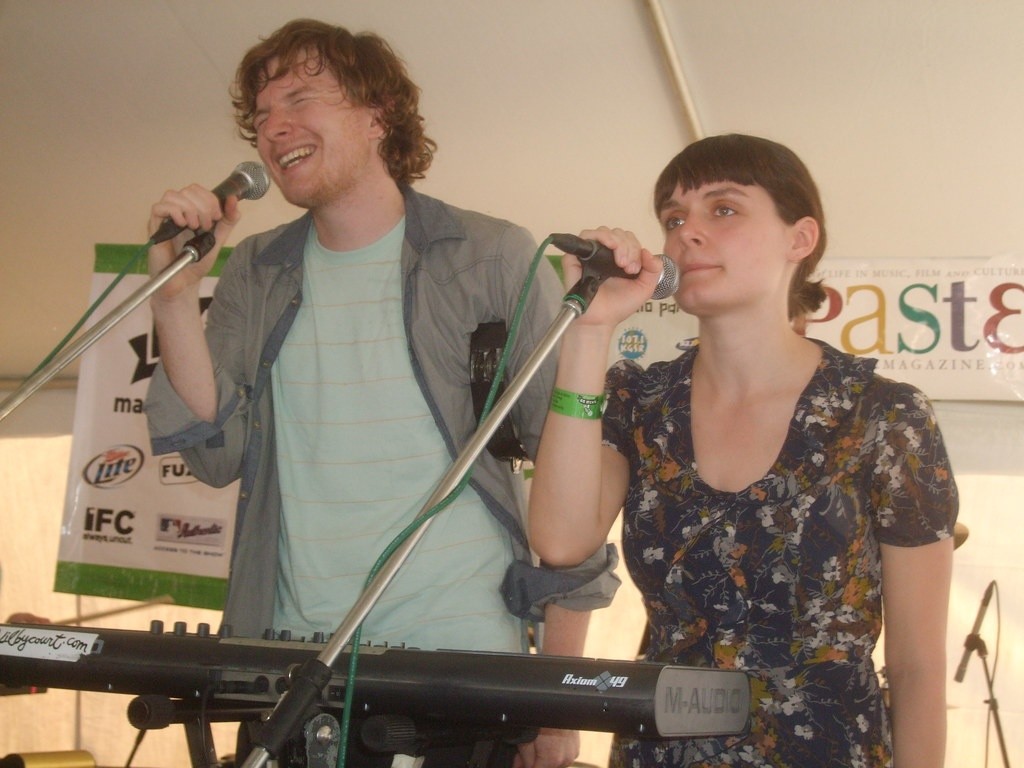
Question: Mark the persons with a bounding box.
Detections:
[147,18,623,768]
[528,136,958,768]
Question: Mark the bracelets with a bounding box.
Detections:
[548,386,605,420]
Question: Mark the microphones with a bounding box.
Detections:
[550,233,680,300]
[152,161,271,245]
[954,584,994,683]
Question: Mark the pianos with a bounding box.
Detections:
[0,616,754,745]
